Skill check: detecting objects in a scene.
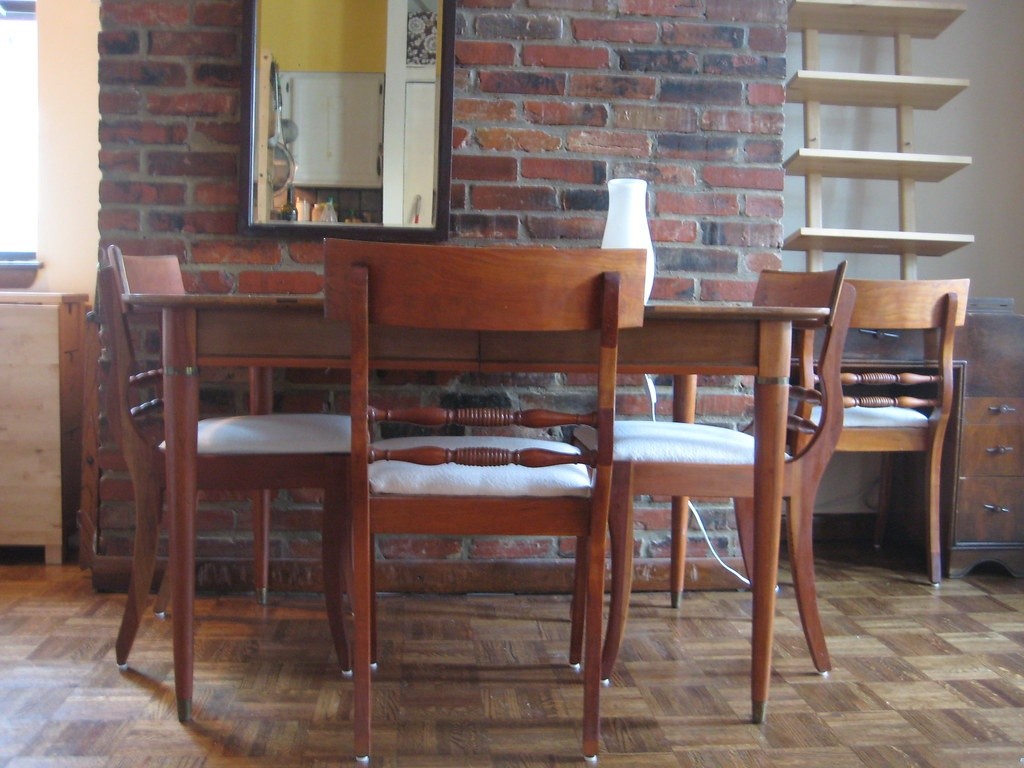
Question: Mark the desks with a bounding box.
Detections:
[120,291,831,724]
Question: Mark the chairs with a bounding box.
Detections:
[789,278,970,586]
[96,242,369,680]
[344,238,646,766]
[572,262,857,689]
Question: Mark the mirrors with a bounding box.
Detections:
[238,0,457,240]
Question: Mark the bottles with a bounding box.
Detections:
[601,178,655,304]
[317,197,338,223]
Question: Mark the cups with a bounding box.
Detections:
[296,197,311,221]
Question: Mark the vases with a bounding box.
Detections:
[601,179,655,304]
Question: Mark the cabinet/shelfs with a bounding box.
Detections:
[786,296,1024,581]
[783,0,974,278]
[276,70,385,190]
[1,291,89,565]
